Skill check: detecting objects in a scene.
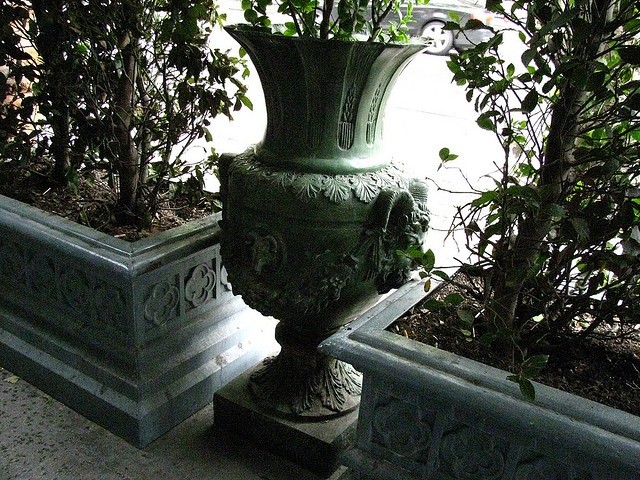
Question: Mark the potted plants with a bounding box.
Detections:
[317,2,640,479]
[0,0,253,450]
[210,0,431,478]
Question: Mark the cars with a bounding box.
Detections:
[317,0,504,56]
